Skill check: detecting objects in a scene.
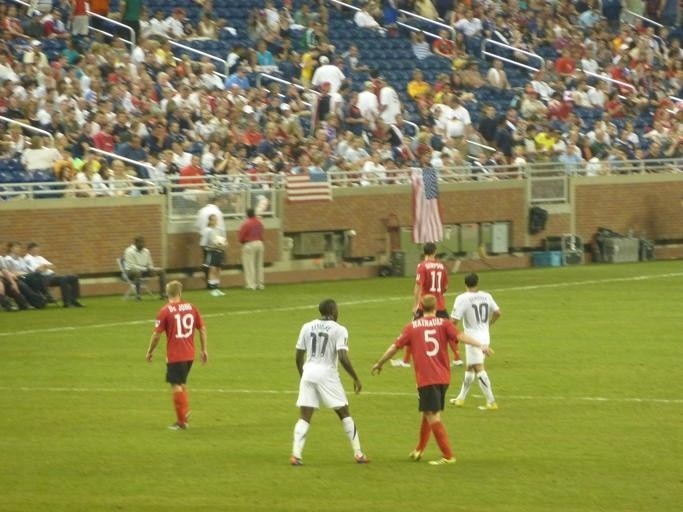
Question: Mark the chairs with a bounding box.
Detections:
[116,256,157,301]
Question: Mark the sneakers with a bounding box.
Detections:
[64,302,71,307]
[409,448,424,460]
[210,290,218,296]
[451,360,464,366]
[355,454,369,463]
[257,283,263,290]
[478,402,498,409]
[185,412,192,422]
[168,422,190,430]
[20,303,33,308]
[390,359,411,367]
[290,456,304,465]
[450,399,464,406]
[245,284,255,290]
[136,295,143,301]
[8,306,19,312]
[217,289,226,296]
[428,457,456,465]
[71,299,84,308]
[161,294,167,298]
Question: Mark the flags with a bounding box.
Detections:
[408,164,446,246]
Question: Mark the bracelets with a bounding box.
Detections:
[478,342,485,351]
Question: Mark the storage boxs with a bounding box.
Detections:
[531,249,562,267]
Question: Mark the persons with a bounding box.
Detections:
[0,253,35,313]
[447,271,502,413]
[2,1,682,203]
[199,214,226,297]
[369,293,494,466]
[3,240,58,309]
[120,235,170,303]
[195,193,226,289]
[237,208,265,291]
[387,241,463,368]
[288,298,372,467]
[22,243,89,308]
[145,280,209,432]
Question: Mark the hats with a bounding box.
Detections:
[240,105,254,115]
[363,80,376,88]
[279,102,291,112]
[30,41,40,46]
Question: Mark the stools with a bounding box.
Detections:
[45,285,62,305]
